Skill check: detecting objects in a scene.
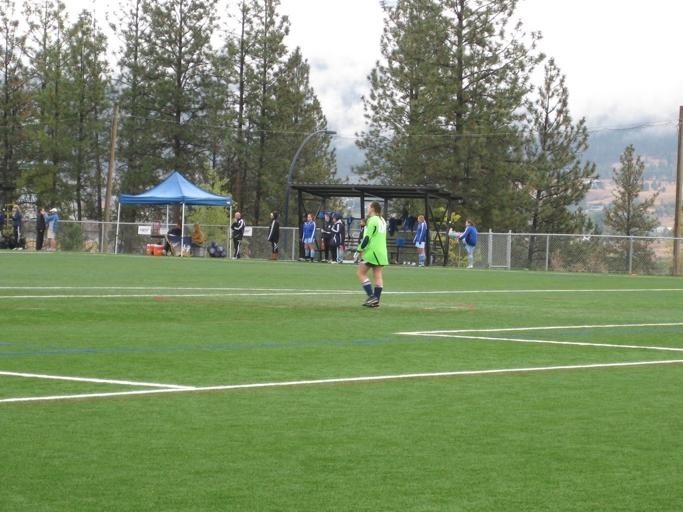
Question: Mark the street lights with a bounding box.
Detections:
[280,129,336,256]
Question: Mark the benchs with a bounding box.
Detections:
[315,239,440,262]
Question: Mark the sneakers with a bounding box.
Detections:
[466,265,473,268]
[231,256,240,260]
[362,296,380,307]
[448,228,453,236]
[298,257,313,262]
[47,248,56,252]
[321,258,343,264]
[12,247,23,251]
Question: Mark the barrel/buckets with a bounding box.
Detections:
[146,243,157,255]
[154,244,166,255]
[192,246,205,256]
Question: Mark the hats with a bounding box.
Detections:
[49,208,57,213]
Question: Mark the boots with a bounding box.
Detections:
[270,254,277,260]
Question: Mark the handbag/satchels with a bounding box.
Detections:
[330,232,343,248]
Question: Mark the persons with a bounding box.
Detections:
[299,212,345,264]
[353,219,367,263]
[448,220,477,269]
[413,214,428,267]
[267,211,280,260]
[231,212,245,260]
[353,202,388,307]
[164,224,204,256]
[36,208,58,251]
[11,205,23,251]
